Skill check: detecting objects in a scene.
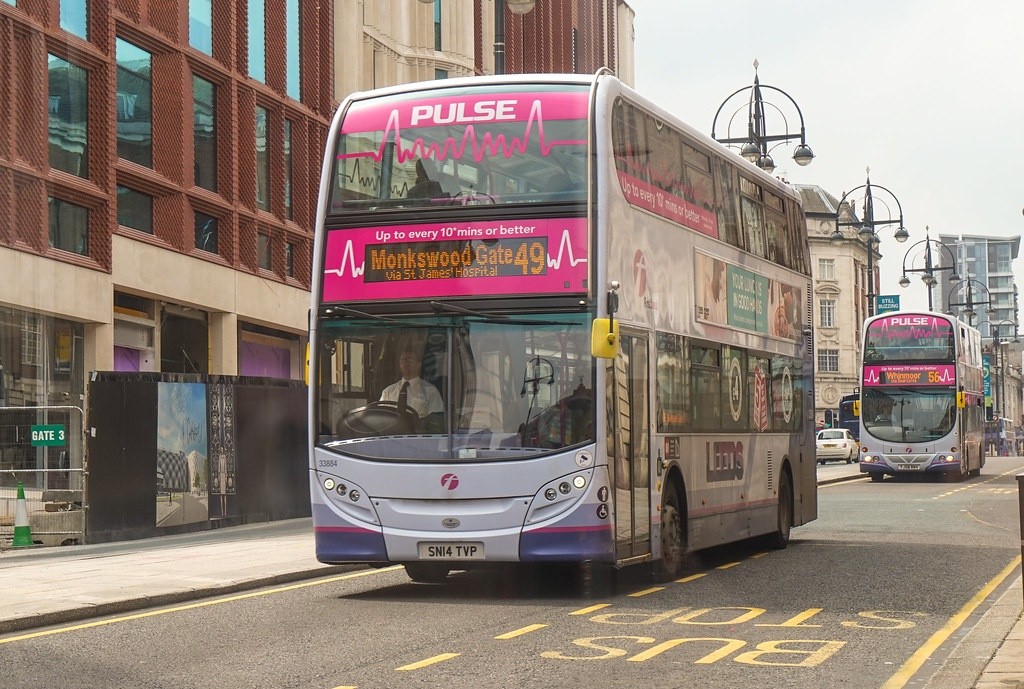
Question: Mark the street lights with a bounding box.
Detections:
[828,164,909,316]
[974,318,1021,457]
[711,59,816,176]
[946,275,996,328]
[899,225,961,312]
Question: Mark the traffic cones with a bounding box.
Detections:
[10,480,36,547]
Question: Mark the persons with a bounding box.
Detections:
[379,350,444,431]
[706,259,726,323]
[876,406,897,422]
[544,173,573,201]
[407,158,452,205]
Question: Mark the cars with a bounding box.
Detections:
[815,428,860,465]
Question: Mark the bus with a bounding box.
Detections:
[853,309,988,483]
[303,65,820,587]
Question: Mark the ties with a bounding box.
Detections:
[397,381,410,411]
